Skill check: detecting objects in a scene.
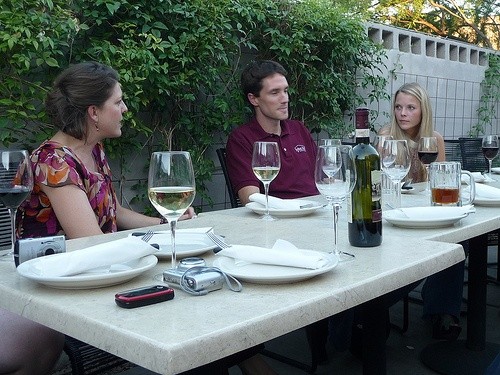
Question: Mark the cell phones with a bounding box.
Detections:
[114,284,175,309]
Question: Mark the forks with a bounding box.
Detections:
[205,232,230,249]
[141,229,154,243]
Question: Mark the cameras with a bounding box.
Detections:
[163,265,223,295]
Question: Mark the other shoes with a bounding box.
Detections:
[428,317,461,340]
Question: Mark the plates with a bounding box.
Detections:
[213,249,338,285]
[461,192,500,208]
[245,198,326,218]
[17,252,158,290]
[382,206,476,229]
[138,235,225,260]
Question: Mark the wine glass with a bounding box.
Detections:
[374,136,398,173]
[417,136,439,194]
[314,145,356,263]
[0,148,34,263]
[252,141,281,222]
[481,135,500,182]
[380,139,411,208]
[319,138,343,210]
[347,107,383,248]
[148,150,197,284]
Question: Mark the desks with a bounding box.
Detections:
[0,167,500,375]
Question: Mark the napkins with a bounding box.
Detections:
[216,238,328,270]
[383,203,477,219]
[34,235,160,277]
[463,182,500,199]
[249,192,315,210]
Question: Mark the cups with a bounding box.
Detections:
[429,162,476,207]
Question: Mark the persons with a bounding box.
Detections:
[0,61,279,375]
[225,60,463,354]
